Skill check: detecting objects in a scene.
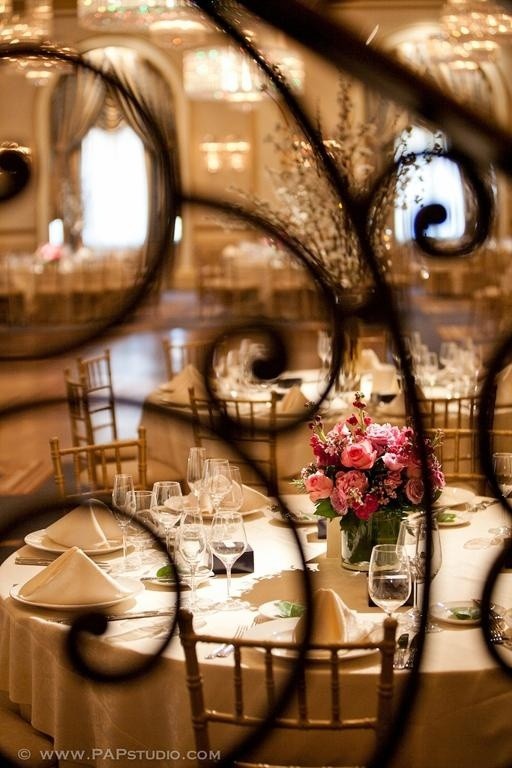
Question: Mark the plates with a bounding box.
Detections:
[432,486,476,508]
[23,527,134,554]
[427,508,474,527]
[427,600,506,625]
[242,615,380,663]
[164,495,267,519]
[272,509,319,524]
[9,573,144,609]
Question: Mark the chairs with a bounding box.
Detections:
[417,392,512,486]
[177,606,398,768]
[50,338,277,511]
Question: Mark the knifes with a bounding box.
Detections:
[392,632,418,670]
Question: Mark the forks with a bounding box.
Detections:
[203,617,261,659]
[470,598,511,652]
[14,553,110,570]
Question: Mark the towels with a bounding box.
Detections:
[293,587,379,658]
[18,498,133,605]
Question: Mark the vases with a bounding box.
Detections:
[339,513,409,573]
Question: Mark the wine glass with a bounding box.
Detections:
[111,446,250,612]
[488,453,512,535]
[367,518,443,655]
[160,332,481,414]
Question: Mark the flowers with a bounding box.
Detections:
[289,391,445,565]
